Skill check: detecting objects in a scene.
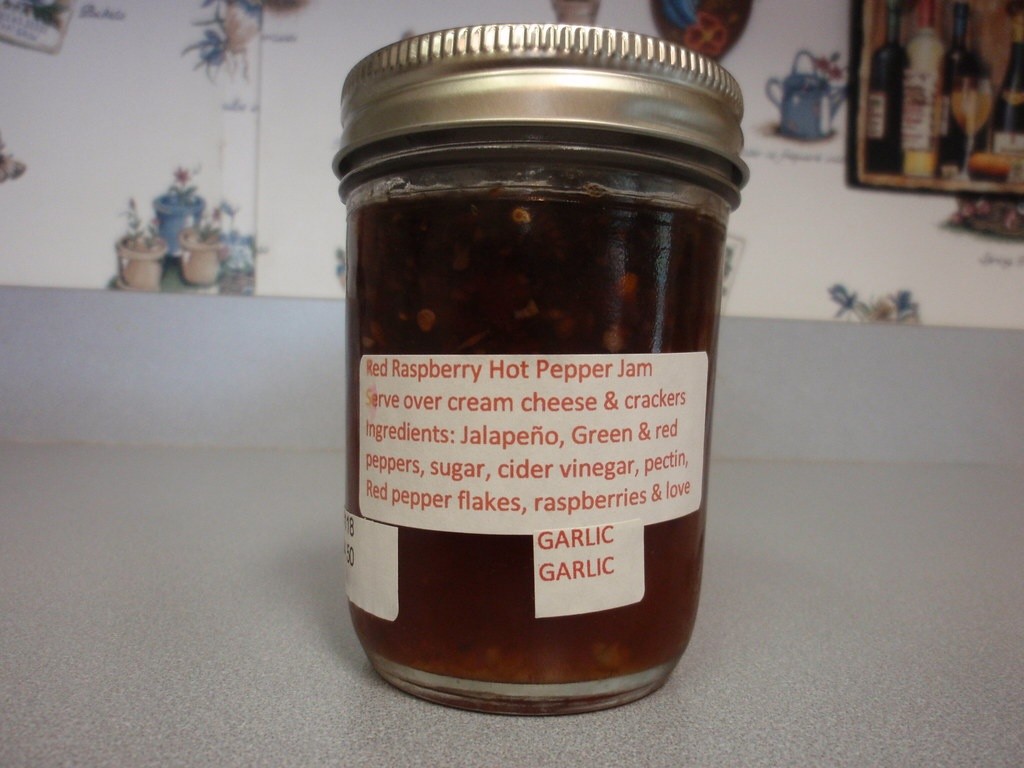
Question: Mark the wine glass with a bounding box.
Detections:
[949,75,994,183]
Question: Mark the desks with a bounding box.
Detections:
[0,287,1024,768]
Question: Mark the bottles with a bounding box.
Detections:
[328,23,752,714]
[863,1,1024,179]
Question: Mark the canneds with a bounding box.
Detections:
[331,22,749,715]
